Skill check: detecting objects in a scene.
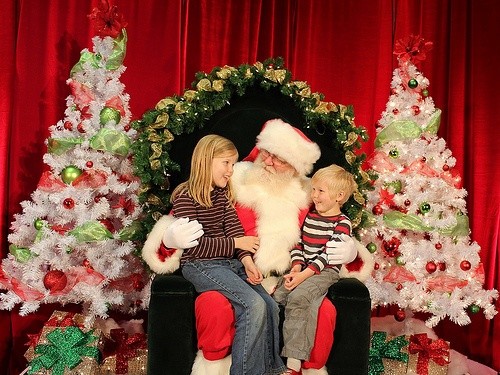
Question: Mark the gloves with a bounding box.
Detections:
[326,233,357,265]
[163,217,204,249]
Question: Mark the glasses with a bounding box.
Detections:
[261,148,287,165]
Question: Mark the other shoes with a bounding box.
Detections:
[277,368,302,375]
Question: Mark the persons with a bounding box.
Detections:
[272,164,353,374]
[170,134,286,375]
[141,120,375,374]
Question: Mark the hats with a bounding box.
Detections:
[241,118,321,176]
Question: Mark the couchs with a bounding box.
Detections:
[147,81,371,375]
